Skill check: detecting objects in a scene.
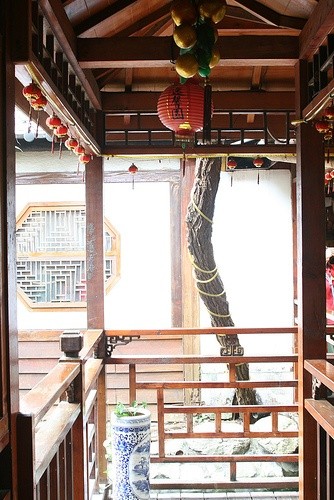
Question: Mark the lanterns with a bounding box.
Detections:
[253,156,265,185]
[226,158,238,186]
[154,77,214,143]
[127,163,137,188]
[314,103,334,195]
[23,81,91,182]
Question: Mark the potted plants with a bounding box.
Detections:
[110,401,151,500]
